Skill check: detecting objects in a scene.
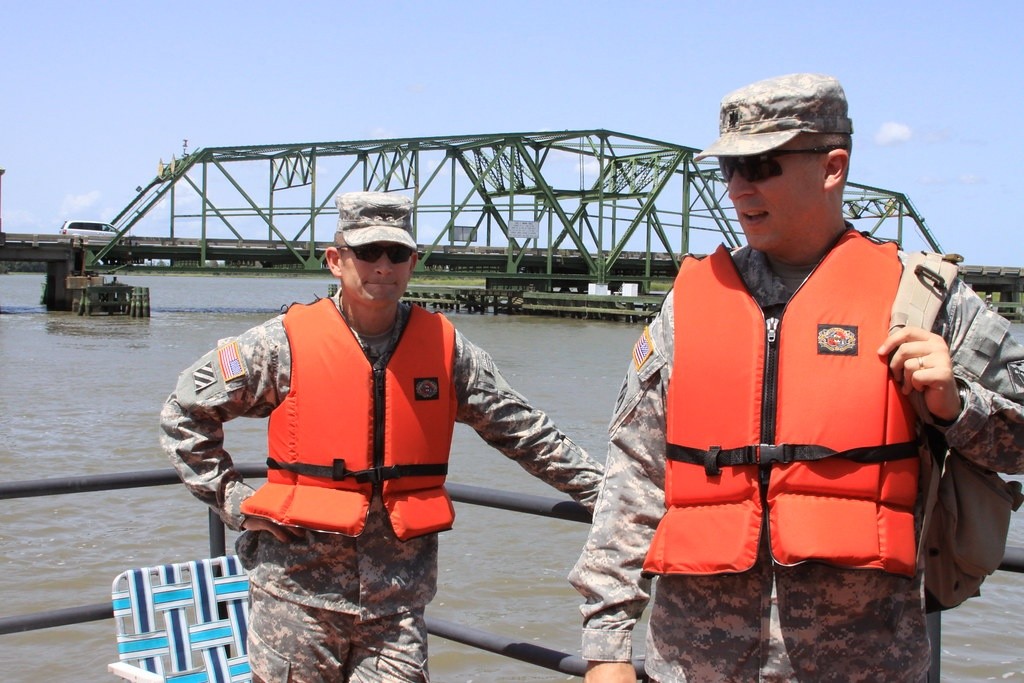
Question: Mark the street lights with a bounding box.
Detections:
[0,166,8,245]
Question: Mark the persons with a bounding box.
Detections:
[567,74,1024,683]
[161,193,606,683]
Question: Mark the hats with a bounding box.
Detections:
[337,193,417,251]
[693,73,853,161]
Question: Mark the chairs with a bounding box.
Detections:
[107,554,252,683]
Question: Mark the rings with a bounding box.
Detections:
[917,356,924,369]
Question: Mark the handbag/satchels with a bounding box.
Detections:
[887,245,1024,612]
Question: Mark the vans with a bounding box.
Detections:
[60,221,125,242]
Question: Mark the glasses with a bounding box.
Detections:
[719,143,847,183]
[337,244,415,265]
